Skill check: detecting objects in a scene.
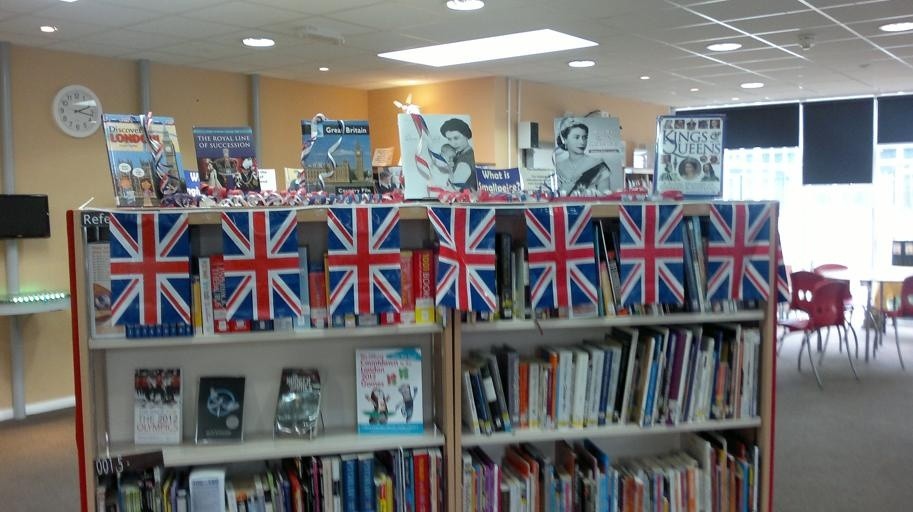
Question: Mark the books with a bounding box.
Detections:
[93,447,442,512]
[133,346,424,445]
[460,216,762,323]
[461,439,759,512]
[84,216,446,329]
[462,330,761,437]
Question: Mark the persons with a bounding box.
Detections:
[379,172,397,192]
[439,118,477,193]
[555,118,613,196]
[682,159,698,180]
[700,162,720,182]
[434,143,457,175]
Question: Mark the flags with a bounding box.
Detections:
[109,212,191,327]
[777,230,789,303]
[706,204,770,301]
[327,208,402,314]
[525,204,598,310]
[427,205,496,313]
[223,209,302,321]
[619,205,684,304]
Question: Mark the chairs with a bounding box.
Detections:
[776,264,913,390]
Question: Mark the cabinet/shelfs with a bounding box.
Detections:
[66,200,779,512]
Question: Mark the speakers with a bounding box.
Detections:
[0,194,51,239]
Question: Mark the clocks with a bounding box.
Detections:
[51,84,103,138]
